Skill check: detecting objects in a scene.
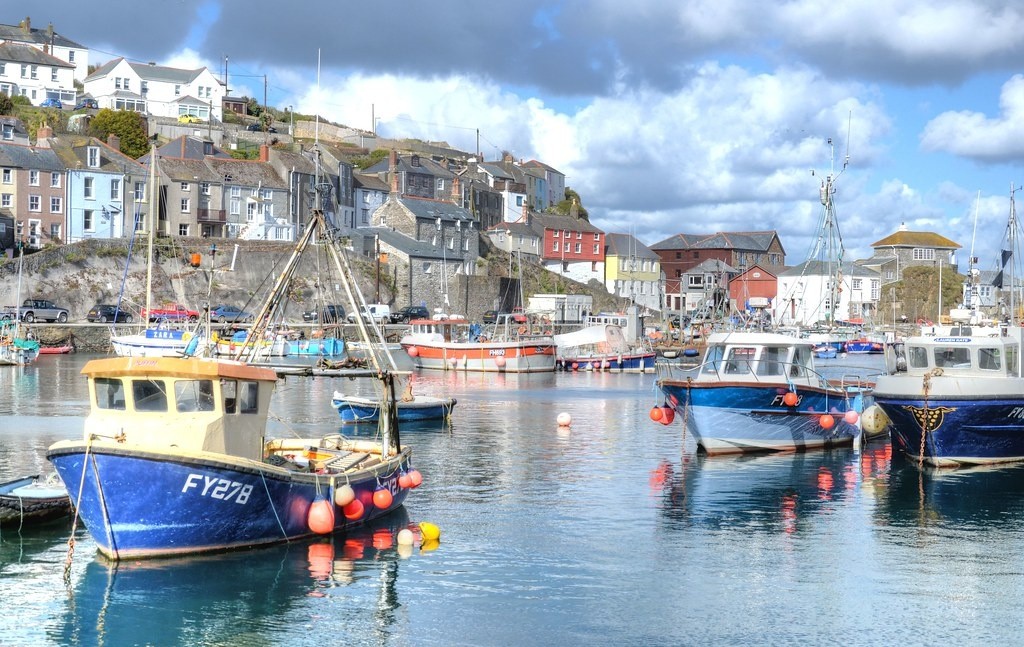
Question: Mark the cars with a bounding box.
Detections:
[203,305,254,323]
[38,98,63,109]
[178,113,202,124]
[0,313,15,322]
[246,122,279,134]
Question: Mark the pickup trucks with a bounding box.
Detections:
[140,303,200,322]
[3,299,70,323]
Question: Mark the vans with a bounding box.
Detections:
[950,309,986,325]
[347,304,390,324]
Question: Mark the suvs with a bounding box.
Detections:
[87,304,133,323]
[302,305,346,324]
[391,306,430,325]
[72,99,99,112]
[483,310,508,323]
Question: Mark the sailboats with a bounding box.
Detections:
[104,145,217,358]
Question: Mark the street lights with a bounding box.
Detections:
[289,105,294,128]
[374,117,382,136]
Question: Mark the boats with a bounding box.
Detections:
[210,328,346,360]
[550,324,658,375]
[330,388,457,423]
[0,473,75,527]
[42,504,413,647]
[652,108,895,456]
[42,45,422,562]
[0,318,41,366]
[816,346,837,360]
[35,345,74,355]
[845,339,885,354]
[396,312,560,373]
[869,179,1024,470]
